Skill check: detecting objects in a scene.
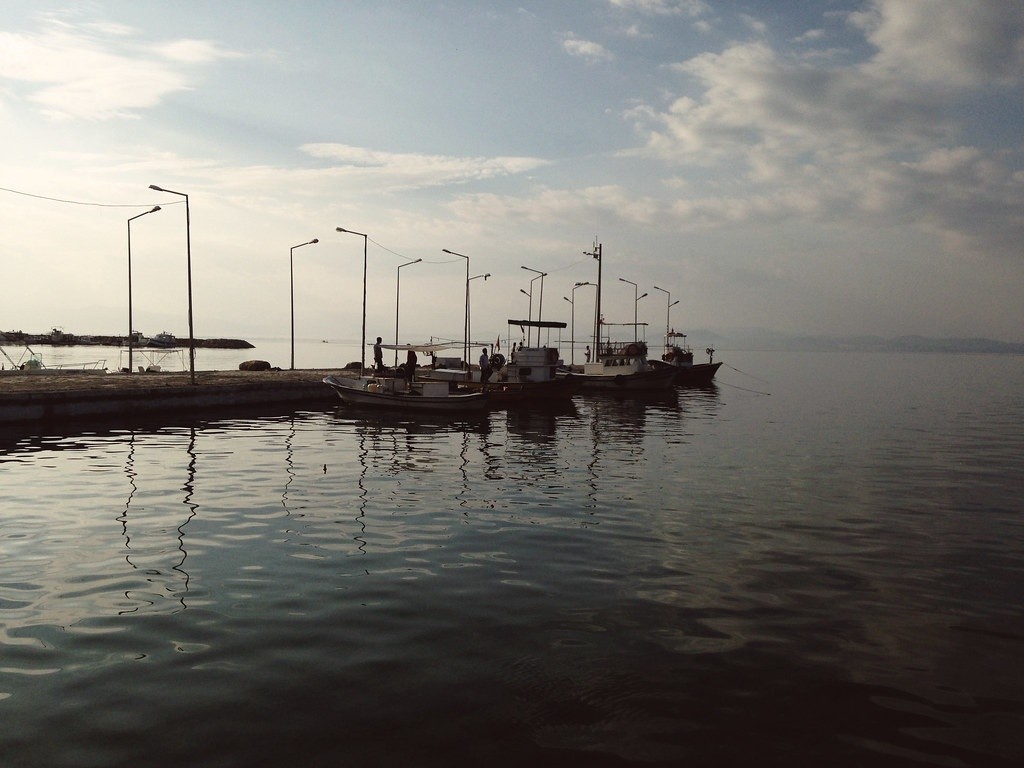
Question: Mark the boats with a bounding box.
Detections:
[326,344,535,424]
[660,327,723,384]
[419,345,583,410]
[552,341,684,394]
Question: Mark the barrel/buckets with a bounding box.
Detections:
[473,372,481,382]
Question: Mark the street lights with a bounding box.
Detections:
[564,282,589,365]
[520,265,543,349]
[335,227,367,375]
[442,249,471,373]
[149,185,195,384]
[291,239,320,369]
[395,258,423,368]
[619,278,648,345]
[521,273,548,347]
[653,286,680,354]
[463,273,491,371]
[127,206,161,372]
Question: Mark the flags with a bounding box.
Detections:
[496,336,500,352]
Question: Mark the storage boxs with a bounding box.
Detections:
[585,363,604,375]
[355,368,474,397]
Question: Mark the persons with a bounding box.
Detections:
[374,337,382,374]
[404,344,417,390]
[511,342,516,353]
[517,342,522,351]
[585,345,590,363]
[480,348,488,387]
